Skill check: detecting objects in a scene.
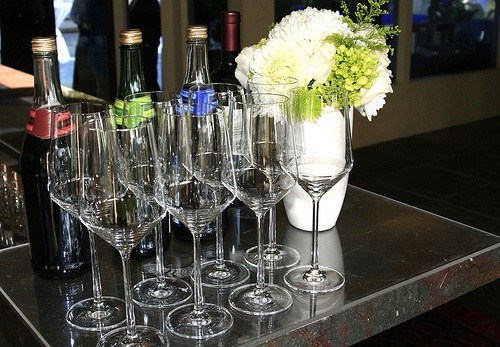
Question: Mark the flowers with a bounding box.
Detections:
[235,0,402,121]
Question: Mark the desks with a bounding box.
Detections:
[0,184,500,347]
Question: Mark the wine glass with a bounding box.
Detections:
[187,82,250,288]
[222,93,293,316]
[121,91,193,309]
[284,86,354,293]
[0,162,29,250]
[243,76,300,270]
[49,103,127,331]
[78,116,170,347]
[159,103,239,340]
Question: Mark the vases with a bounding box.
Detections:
[281,104,354,232]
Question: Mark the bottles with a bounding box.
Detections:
[209,11,259,226]
[112,30,167,259]
[171,27,229,245]
[20,37,92,280]
